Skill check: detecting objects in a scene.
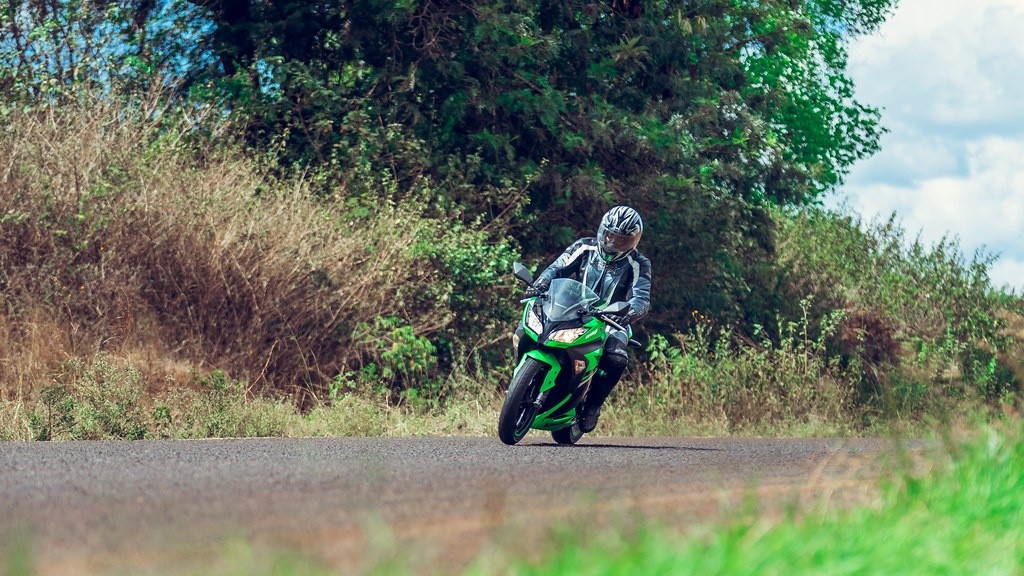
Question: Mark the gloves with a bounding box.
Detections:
[606,314,631,332]
[525,282,549,299]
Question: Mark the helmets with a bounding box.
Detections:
[597,205,644,265]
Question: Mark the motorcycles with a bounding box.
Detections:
[498,262,641,445]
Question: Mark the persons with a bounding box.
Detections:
[511,204,652,434]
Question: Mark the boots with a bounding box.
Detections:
[579,352,629,432]
